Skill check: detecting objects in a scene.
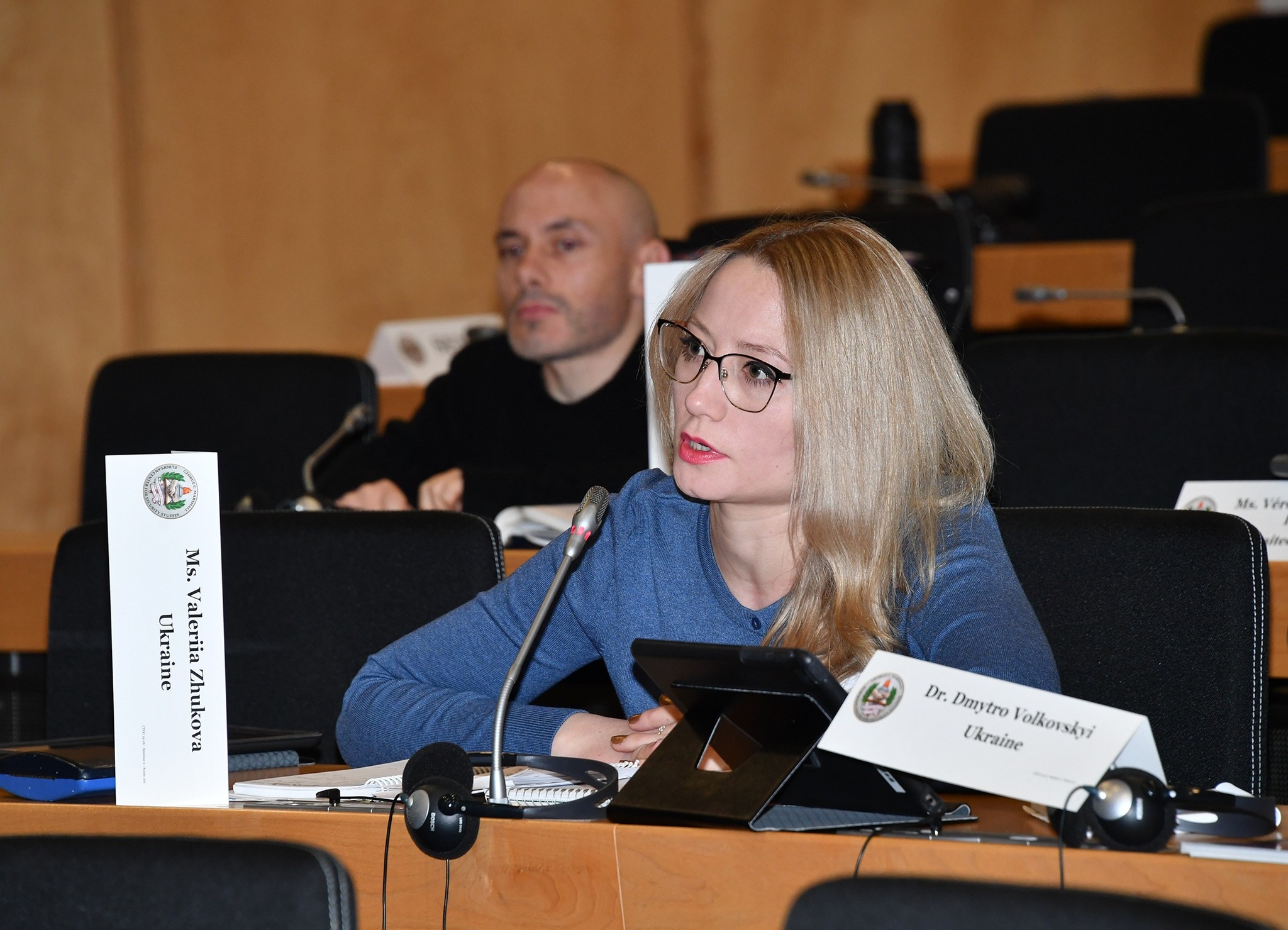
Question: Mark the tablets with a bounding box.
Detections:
[627,640,943,817]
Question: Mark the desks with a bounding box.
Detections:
[0,753,1288,929]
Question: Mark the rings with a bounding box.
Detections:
[658,726,665,735]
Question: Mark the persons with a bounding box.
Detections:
[334,217,1062,766]
[311,157,670,521]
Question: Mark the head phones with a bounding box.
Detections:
[402,740,618,858]
[1048,768,1276,853]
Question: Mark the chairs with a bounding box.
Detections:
[0,7,1288,930]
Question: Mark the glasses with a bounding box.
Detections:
[656,316,794,415]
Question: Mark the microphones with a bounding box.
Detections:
[478,486,612,817]
[803,169,951,212]
[302,404,377,495]
[1017,286,1187,326]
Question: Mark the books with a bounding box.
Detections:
[232,758,639,807]
